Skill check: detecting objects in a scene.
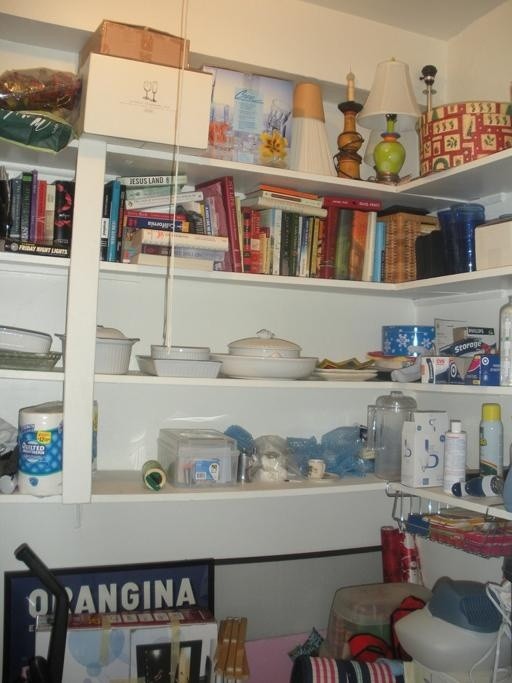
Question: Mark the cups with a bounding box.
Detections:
[309,458,328,479]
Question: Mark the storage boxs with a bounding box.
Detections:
[70,19,215,158]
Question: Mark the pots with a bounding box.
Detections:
[55,326,141,376]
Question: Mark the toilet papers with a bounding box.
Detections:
[141,459,166,491]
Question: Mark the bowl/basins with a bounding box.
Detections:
[368,352,418,370]
[149,342,211,359]
[1,348,61,369]
[135,352,224,379]
[228,328,303,358]
[1,325,52,353]
[208,348,318,380]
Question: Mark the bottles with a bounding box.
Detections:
[441,418,466,499]
[236,447,260,482]
[451,475,504,498]
[480,403,507,476]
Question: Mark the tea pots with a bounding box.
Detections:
[367,388,415,481]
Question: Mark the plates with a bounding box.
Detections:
[316,366,378,382]
[302,471,338,482]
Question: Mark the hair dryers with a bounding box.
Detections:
[465,474,505,498]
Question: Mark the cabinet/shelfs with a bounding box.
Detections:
[0,136,512,574]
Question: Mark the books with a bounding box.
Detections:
[4,169,75,259]
[100,172,386,285]
